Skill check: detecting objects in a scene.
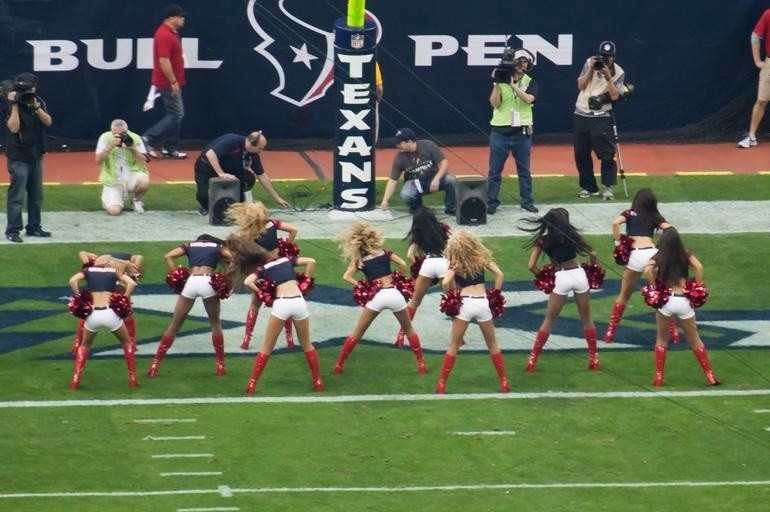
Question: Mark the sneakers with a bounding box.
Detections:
[29,229,51,237]
[142,137,158,158]
[7,233,22,242]
[522,203,538,213]
[487,206,495,213]
[577,188,599,198]
[160,148,188,159]
[603,186,614,201]
[200,205,208,215]
[738,136,757,148]
[131,200,144,214]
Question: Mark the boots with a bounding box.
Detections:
[398,307,426,376]
[333,336,357,375]
[244,308,268,394]
[285,317,322,392]
[525,330,551,374]
[435,352,455,395]
[491,353,509,393]
[213,335,226,377]
[605,303,625,343]
[586,329,601,372]
[68,316,88,388]
[148,336,175,376]
[652,317,719,388]
[124,315,138,392]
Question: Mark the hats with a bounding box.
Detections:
[165,5,187,17]
[514,50,533,62]
[390,128,414,144]
[18,74,39,87]
[598,41,615,55]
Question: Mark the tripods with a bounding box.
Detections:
[609,101,629,199]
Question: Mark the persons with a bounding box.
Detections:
[67,250,143,389]
[573,40,625,200]
[331,223,427,375]
[141,4,189,159]
[435,231,510,394]
[604,188,680,345]
[2,72,53,242]
[95,118,150,216]
[643,226,720,387]
[193,131,292,215]
[737,8,770,149]
[395,207,451,348]
[225,201,323,393]
[486,48,539,214]
[147,233,233,379]
[526,209,600,372]
[381,127,459,215]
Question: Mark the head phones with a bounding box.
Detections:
[515,48,536,74]
[251,128,263,147]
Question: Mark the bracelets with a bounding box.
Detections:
[171,80,177,84]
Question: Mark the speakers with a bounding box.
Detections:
[454,175,489,226]
[207,176,241,226]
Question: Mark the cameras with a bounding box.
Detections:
[593,53,605,70]
[119,131,134,147]
